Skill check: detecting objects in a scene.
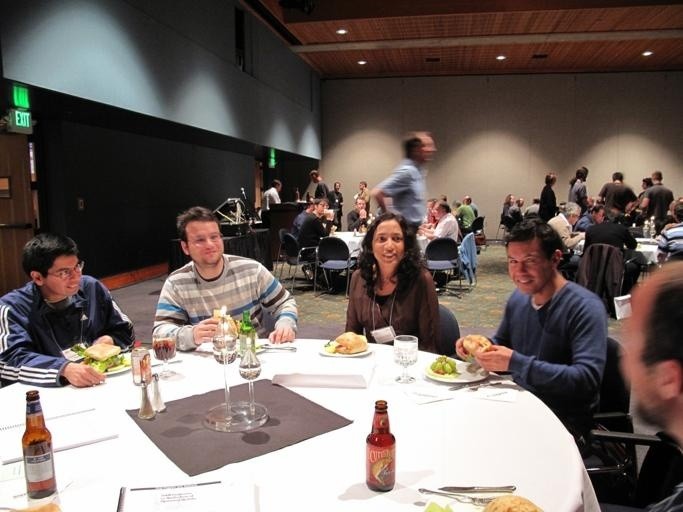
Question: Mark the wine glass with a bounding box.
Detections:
[150,331,177,379]
[392,335,417,384]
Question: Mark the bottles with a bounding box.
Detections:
[364,400,396,492]
[305,192,309,202]
[137,373,166,420]
[20,388,55,498]
[295,187,299,202]
[238,312,256,356]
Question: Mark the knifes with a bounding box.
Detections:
[449,375,500,390]
[441,485,516,492]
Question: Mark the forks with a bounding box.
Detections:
[258,343,296,351]
[417,485,496,507]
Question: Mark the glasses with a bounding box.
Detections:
[49,260,84,278]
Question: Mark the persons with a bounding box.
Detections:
[597,260,683,511]
[344,210,440,355]
[347,197,369,231]
[0,232,136,389]
[372,132,435,234]
[568,167,594,210]
[597,172,638,213]
[329,182,344,231]
[456,220,607,462]
[576,206,605,232]
[309,170,330,199]
[417,195,478,288]
[152,206,298,352]
[503,194,540,220]
[537,175,559,220]
[635,172,683,261]
[358,181,369,209]
[583,208,642,296]
[293,198,335,290]
[546,202,586,270]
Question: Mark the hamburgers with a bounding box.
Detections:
[483,495,543,512]
[327,331,369,354]
[80,342,127,374]
[463,335,493,356]
[216,314,240,340]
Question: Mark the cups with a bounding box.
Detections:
[326,210,333,220]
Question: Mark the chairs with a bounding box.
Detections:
[273,197,487,297]
[491,196,683,323]
[437,298,465,360]
[574,332,681,511]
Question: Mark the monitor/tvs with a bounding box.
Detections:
[212,198,240,220]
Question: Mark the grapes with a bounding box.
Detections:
[431,354,457,375]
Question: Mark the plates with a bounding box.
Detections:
[256,339,266,355]
[427,362,487,383]
[102,352,140,376]
[319,347,371,357]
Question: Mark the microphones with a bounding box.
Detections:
[240,187,247,200]
